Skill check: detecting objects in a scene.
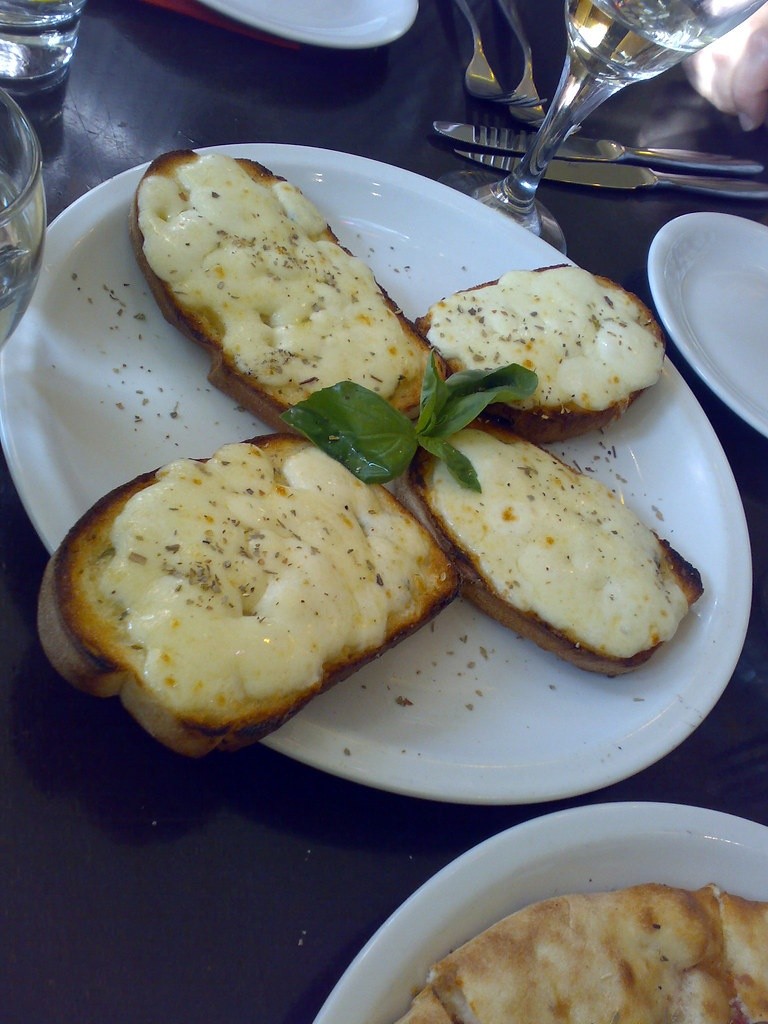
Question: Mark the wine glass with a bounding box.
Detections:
[423,0,768,257]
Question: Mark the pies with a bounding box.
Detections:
[387,883,768,1024]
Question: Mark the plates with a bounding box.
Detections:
[198,0,420,50]
[0,142,753,808]
[313,798,767,1021]
[646,211,768,437]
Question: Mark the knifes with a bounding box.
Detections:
[450,145,767,203]
[433,119,763,174]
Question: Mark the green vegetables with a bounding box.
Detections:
[280,349,540,493]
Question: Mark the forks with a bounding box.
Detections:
[455,0,580,133]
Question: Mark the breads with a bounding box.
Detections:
[412,264,667,444]
[392,418,705,677]
[130,149,450,436]
[35,435,461,756]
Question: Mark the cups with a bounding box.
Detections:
[0,88,47,353]
[0,0,86,96]
